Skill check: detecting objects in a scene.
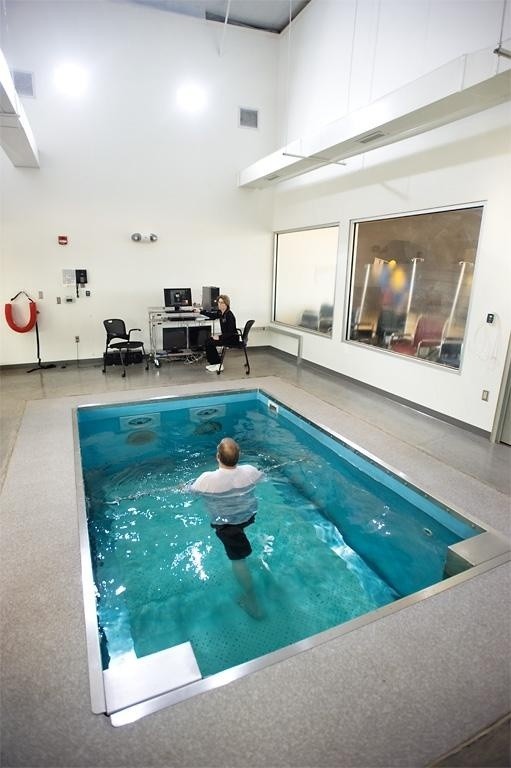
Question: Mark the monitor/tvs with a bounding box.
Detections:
[164,288,192,313]
[188,326,211,351]
[163,327,187,353]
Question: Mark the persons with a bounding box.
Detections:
[193,295,239,372]
[190,438,266,619]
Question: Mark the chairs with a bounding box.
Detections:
[216,319,254,374]
[387,312,449,357]
[103,319,149,377]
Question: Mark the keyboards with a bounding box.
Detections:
[168,317,195,321]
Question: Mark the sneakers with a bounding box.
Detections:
[206,363,224,372]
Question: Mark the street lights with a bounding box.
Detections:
[403,257,425,330]
[446,260,474,337]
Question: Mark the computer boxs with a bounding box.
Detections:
[202,287,219,311]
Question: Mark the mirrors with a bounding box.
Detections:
[272,222,340,340]
[340,200,486,374]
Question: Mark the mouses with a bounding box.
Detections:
[197,317,204,320]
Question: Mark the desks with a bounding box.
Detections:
[149,305,222,368]
